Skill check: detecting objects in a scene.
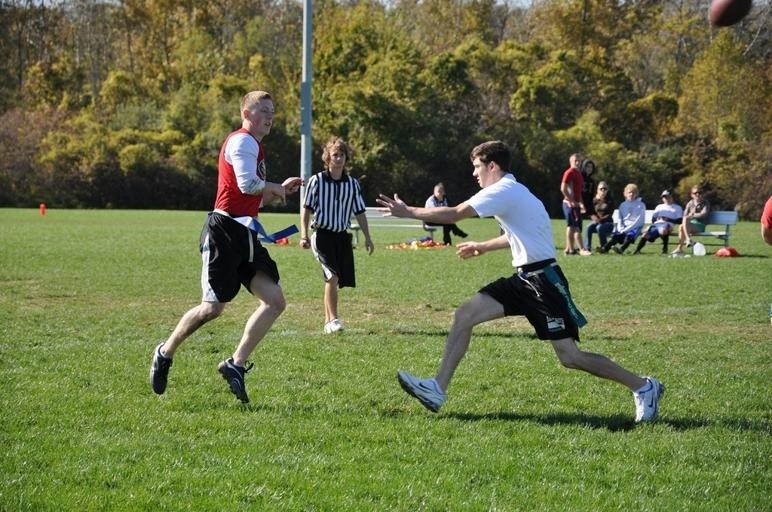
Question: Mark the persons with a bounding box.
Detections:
[376,139,667,428]
[584,180,616,252]
[629,188,684,255]
[670,183,712,257]
[597,182,648,254]
[560,151,594,256]
[760,195,772,247]
[423,182,469,246]
[147,88,306,404]
[580,158,597,220]
[298,135,375,335]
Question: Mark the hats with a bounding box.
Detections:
[660,189,672,197]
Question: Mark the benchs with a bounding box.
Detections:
[342,204,440,242]
[638,208,740,252]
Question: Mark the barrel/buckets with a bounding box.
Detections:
[693,241,706,256]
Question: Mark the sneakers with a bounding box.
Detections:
[148,342,173,397]
[685,239,697,247]
[456,231,468,238]
[661,248,668,254]
[671,249,684,254]
[443,239,452,246]
[561,240,623,255]
[631,251,642,255]
[323,318,345,335]
[216,356,255,406]
[633,375,667,424]
[395,369,449,413]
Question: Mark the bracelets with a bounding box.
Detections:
[299,236,310,242]
